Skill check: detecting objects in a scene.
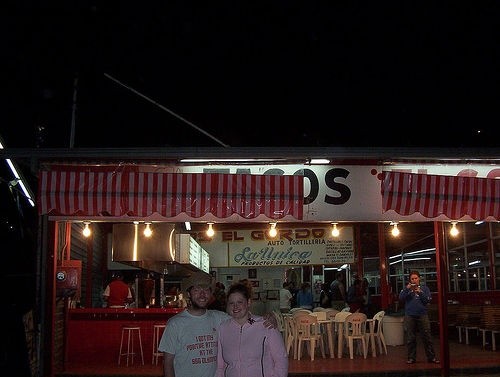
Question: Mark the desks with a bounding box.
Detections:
[318,320,377,359]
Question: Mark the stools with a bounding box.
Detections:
[117,326,144,367]
[152,324,167,367]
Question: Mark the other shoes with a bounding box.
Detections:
[406,358,415,364]
[429,357,440,364]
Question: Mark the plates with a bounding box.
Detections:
[110,306,125,309]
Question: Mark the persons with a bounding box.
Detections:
[158,270,278,377]
[207,282,228,313]
[102,270,133,309]
[320,271,368,313]
[400,271,440,364]
[279,281,313,313]
[216,279,289,377]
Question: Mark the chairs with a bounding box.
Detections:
[272,307,387,362]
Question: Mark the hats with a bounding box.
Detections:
[181,272,217,294]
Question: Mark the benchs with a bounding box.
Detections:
[430,304,500,351]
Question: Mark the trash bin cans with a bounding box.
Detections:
[382,310,405,347]
